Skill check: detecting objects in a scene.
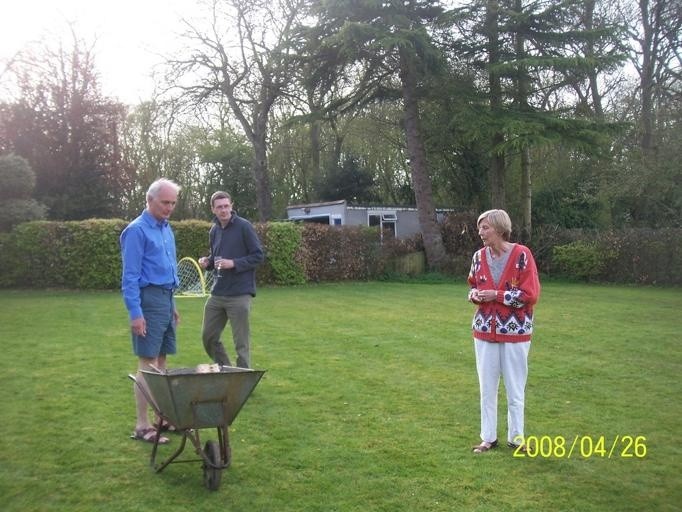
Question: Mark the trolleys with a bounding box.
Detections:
[126,361,267,492]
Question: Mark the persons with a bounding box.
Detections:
[198,190,264,369]
[119,177,192,445]
[467,207,542,457]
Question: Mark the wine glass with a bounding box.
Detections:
[214,256,223,278]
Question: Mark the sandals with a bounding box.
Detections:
[130,427,170,443]
[473,439,498,453]
[153,421,193,432]
[508,442,533,452]
